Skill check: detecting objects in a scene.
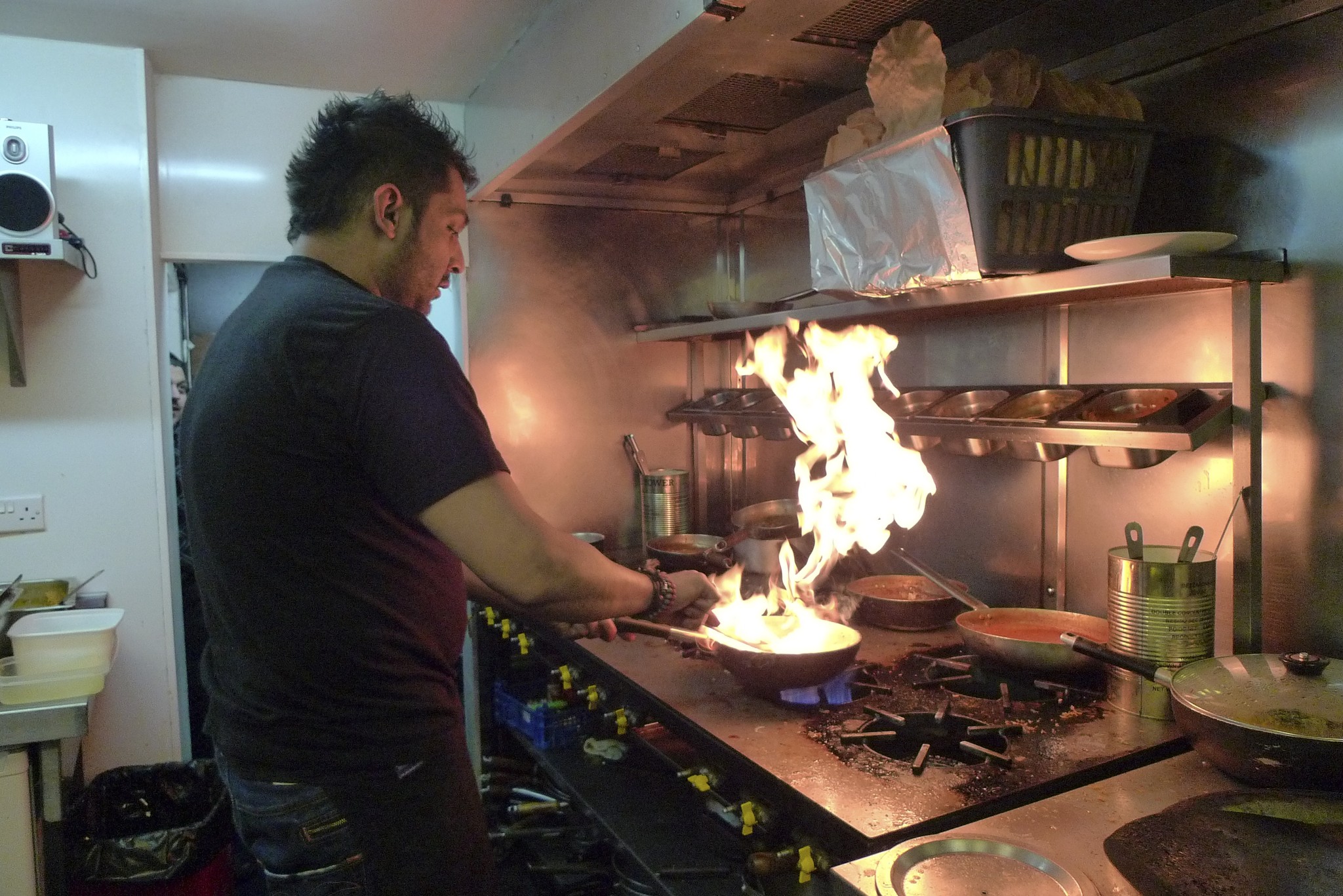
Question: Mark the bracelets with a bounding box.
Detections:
[634,565,677,621]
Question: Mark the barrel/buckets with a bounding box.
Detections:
[640,468,692,556]
[1106,545,1217,720]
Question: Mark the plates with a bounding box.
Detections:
[1064,231,1239,264]
[874,832,1098,896]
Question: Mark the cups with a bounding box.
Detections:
[573,532,605,554]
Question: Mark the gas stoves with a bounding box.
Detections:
[464,561,1192,882]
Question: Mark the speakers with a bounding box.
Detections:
[0,120,59,239]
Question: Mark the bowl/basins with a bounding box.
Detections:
[733,529,807,574]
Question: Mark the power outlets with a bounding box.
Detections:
[0,497,46,532]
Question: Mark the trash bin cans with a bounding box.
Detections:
[63,758,229,896]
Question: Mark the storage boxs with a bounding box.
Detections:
[799,108,1150,272]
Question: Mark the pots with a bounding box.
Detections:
[1061,631,1343,789]
[645,534,733,576]
[609,614,862,690]
[479,755,658,896]
[714,498,801,552]
[886,543,1107,682]
[845,555,970,631]
[706,288,818,320]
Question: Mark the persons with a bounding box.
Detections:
[171,86,722,896]
